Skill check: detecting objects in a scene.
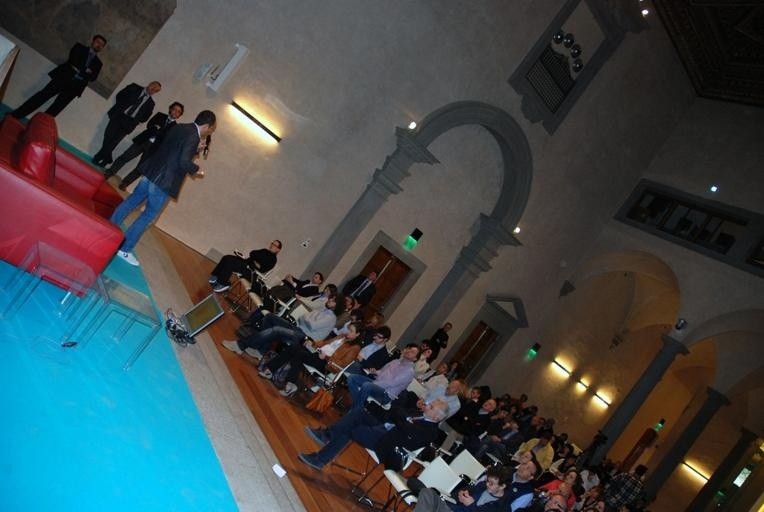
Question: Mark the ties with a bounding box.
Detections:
[126,94,147,116]
[165,118,171,128]
[75,52,94,80]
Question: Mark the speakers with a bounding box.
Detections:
[208,42,249,92]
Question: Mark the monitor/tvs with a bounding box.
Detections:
[181,293,225,338]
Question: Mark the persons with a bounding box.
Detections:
[108,109,217,267]
[206,240,282,293]
[90,79,161,168]
[102,101,185,192]
[5,33,107,125]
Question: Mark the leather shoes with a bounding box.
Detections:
[91,157,127,191]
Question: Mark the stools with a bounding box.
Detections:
[4,240,162,371]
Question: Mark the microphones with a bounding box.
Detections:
[203,134,211,156]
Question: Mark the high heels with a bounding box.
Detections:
[257,369,273,379]
[279,381,298,398]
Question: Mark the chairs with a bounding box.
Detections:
[229,254,645,512]
[0,110,123,297]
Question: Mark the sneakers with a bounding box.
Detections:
[297,425,328,470]
[118,250,141,267]
[222,339,263,360]
[208,274,230,292]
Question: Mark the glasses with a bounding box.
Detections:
[272,242,279,248]
